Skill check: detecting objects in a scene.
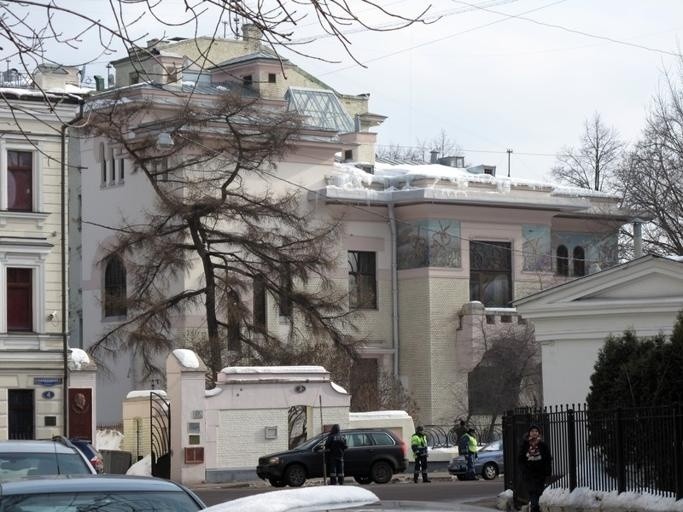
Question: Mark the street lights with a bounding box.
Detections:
[46,90,83,436]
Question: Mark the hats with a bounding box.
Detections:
[529,424,541,435]
[417,426,423,431]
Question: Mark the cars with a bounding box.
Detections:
[448,440,504,480]
[1,435,209,510]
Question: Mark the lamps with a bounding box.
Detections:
[155,133,174,151]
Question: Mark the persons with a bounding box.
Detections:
[456,421,468,455]
[324,424,348,485]
[411,426,432,484]
[524,425,552,512]
[461,428,479,481]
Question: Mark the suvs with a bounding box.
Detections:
[257,428,407,487]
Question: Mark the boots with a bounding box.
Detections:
[422,473,431,482]
[414,473,419,483]
